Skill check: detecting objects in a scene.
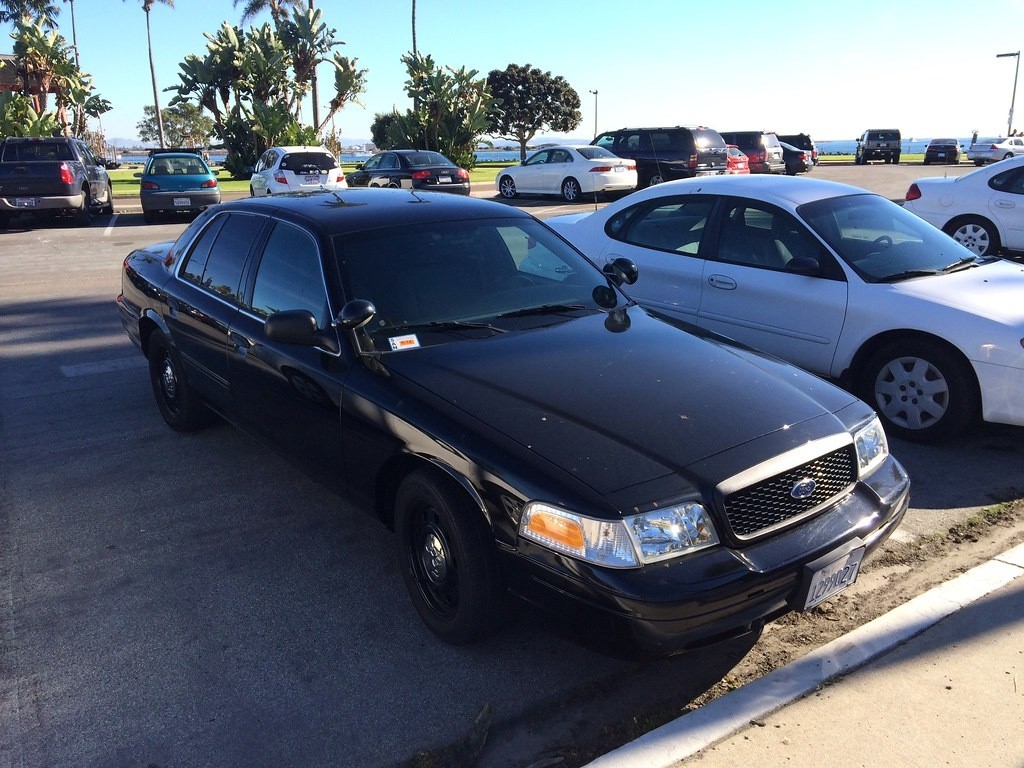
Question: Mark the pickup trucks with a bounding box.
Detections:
[0,136,114,225]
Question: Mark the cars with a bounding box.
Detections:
[345,148,471,196]
[133,151,222,221]
[115,189,914,647]
[779,142,813,177]
[967,135,1024,166]
[724,145,751,175]
[924,139,965,165]
[903,155,1023,256]
[494,145,638,203]
[247,146,349,196]
[523,174,1024,441]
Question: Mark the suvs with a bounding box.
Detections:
[592,128,728,186]
[854,128,901,164]
[779,134,818,165]
[720,132,786,174]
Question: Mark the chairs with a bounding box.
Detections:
[722,218,760,263]
[772,215,806,265]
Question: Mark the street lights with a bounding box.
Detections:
[997,52,1021,135]
[590,91,598,138]
